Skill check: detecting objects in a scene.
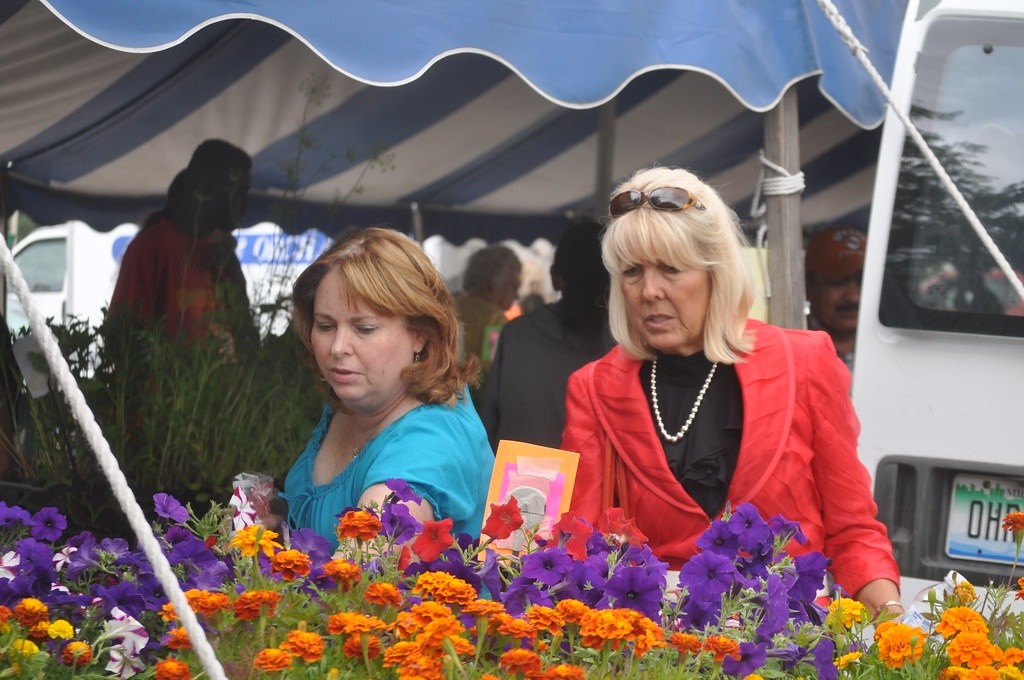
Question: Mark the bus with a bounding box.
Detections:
[851,0,1024,634]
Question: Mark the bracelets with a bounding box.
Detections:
[873,600,905,621]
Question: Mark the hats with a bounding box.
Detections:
[804,227,867,283]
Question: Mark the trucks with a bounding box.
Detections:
[6,219,336,366]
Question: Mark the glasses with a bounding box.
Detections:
[610,186,706,219]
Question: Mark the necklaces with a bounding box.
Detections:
[650,354,717,444]
[351,421,374,461]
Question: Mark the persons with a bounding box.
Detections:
[560,167,906,630]
[805,226,868,370]
[473,222,619,458]
[104,138,259,362]
[218,227,496,562]
[449,245,523,385]
